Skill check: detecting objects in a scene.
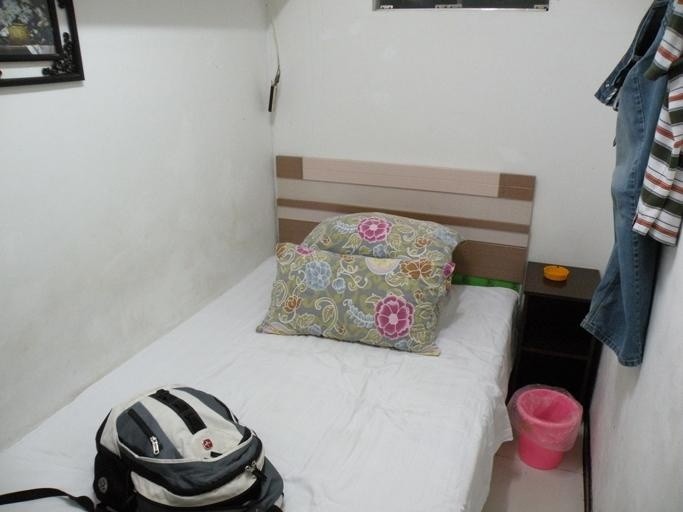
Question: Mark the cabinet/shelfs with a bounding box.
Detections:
[505,262,602,424]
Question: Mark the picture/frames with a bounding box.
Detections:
[0,0,84,87]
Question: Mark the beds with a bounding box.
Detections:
[0,156,536,511]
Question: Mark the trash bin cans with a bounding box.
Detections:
[516,388,583,470]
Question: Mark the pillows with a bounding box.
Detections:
[255,213,466,355]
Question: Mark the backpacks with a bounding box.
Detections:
[87,384,286,512]
